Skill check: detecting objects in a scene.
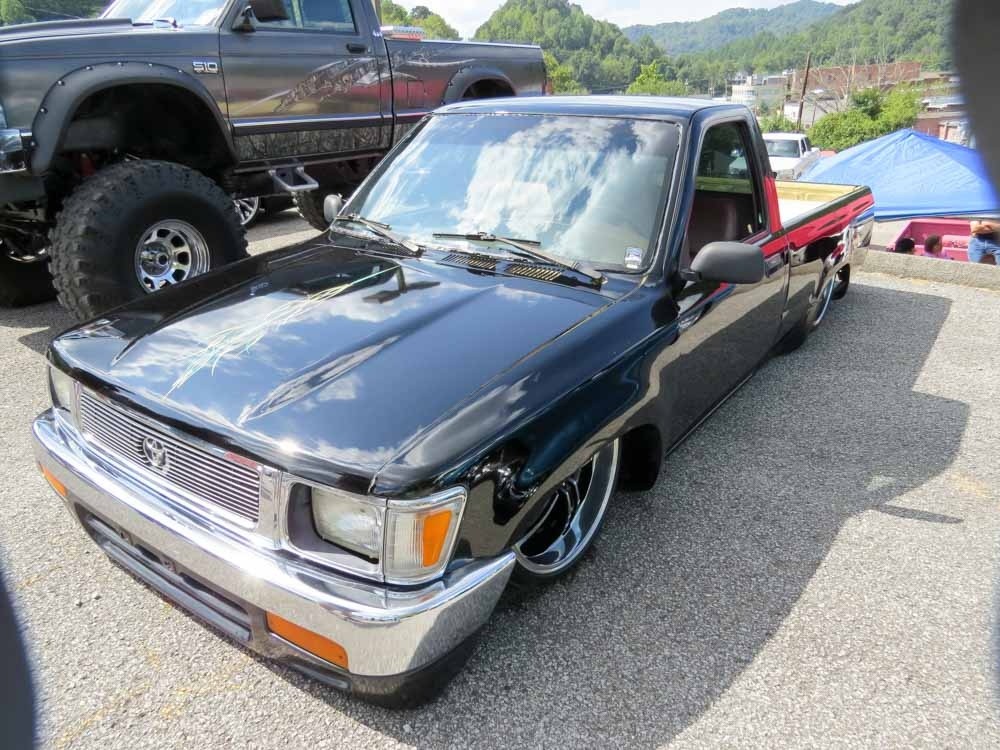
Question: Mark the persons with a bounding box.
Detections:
[922,235,952,259]
[894,238,915,255]
[968,221,1000,265]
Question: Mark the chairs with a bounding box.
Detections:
[688,198,737,258]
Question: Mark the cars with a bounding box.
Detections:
[727,132,822,176]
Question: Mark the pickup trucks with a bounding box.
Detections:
[1,0,553,334]
[31,102,875,702]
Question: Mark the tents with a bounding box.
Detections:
[800,128,1000,223]
